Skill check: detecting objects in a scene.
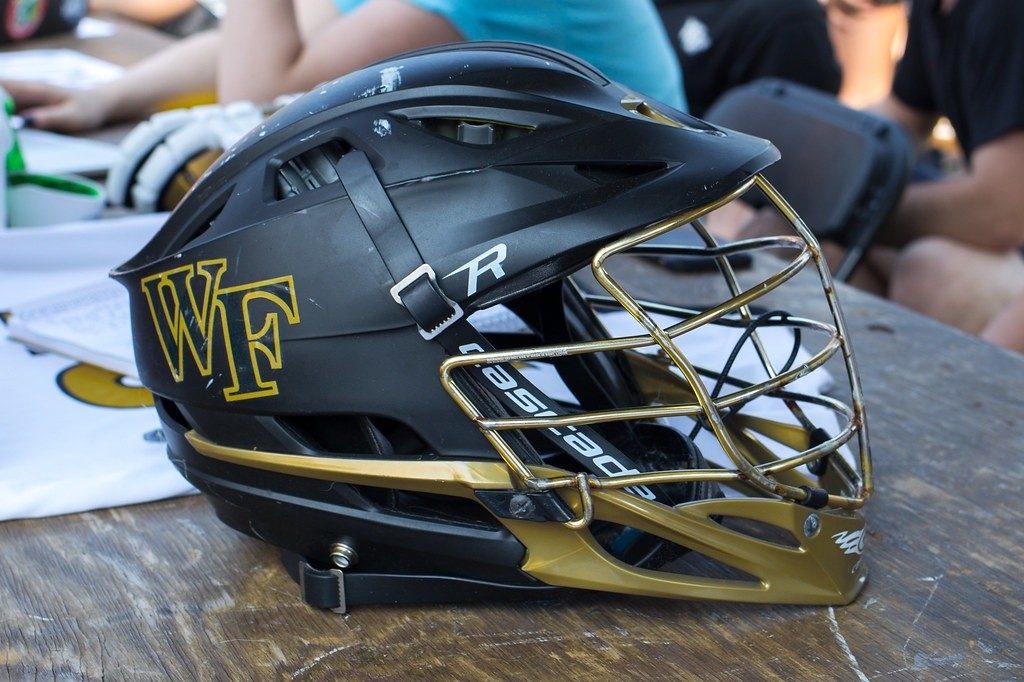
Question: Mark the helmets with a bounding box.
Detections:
[110,39,875,603]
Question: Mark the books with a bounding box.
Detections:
[0,269,137,382]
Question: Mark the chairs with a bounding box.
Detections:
[703,78,912,283]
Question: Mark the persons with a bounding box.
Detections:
[699,0,1024,362]
[0,0,688,134]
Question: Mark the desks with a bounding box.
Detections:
[0,120,1024,682]
